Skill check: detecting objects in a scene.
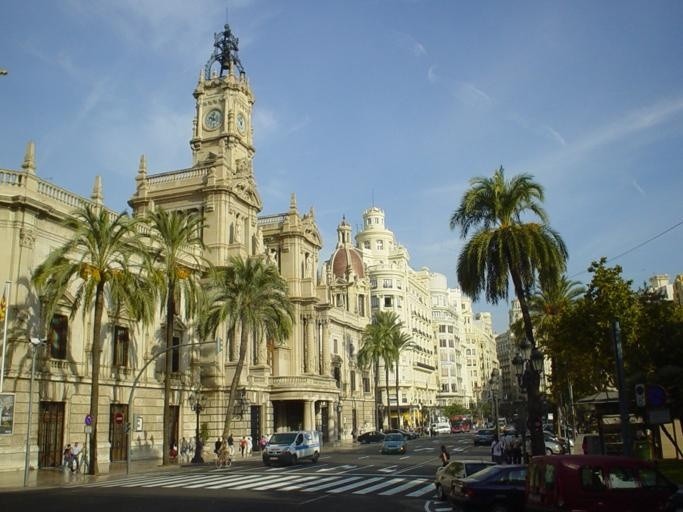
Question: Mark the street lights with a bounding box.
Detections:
[23,337,40,486]
[490,376,500,442]
[512,347,545,464]
[189,394,208,464]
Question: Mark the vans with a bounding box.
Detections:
[262,431,320,465]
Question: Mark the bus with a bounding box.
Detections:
[423,415,473,434]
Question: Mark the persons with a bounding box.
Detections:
[73,442,89,471]
[557,437,570,455]
[489,431,533,481]
[70,442,81,471]
[168,432,273,466]
[439,445,451,465]
[60,443,75,473]
[349,422,438,440]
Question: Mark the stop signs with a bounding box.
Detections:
[115,413,123,424]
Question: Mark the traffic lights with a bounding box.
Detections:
[216,336,223,353]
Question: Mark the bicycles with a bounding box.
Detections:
[215,451,231,469]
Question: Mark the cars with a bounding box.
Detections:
[582,432,630,455]
[474,417,577,456]
[357,428,416,455]
[434,455,683,512]
